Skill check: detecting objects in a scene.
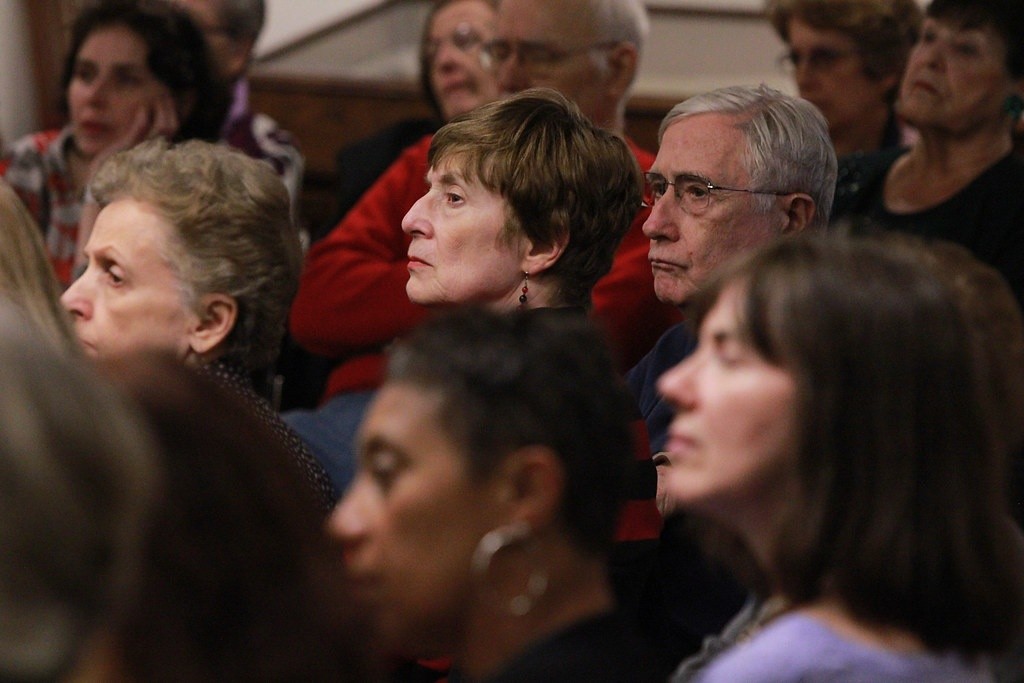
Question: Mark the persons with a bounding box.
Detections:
[0,0,1024,683]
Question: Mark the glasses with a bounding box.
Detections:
[478,39,619,76]
[640,170,792,213]
[775,47,863,74]
